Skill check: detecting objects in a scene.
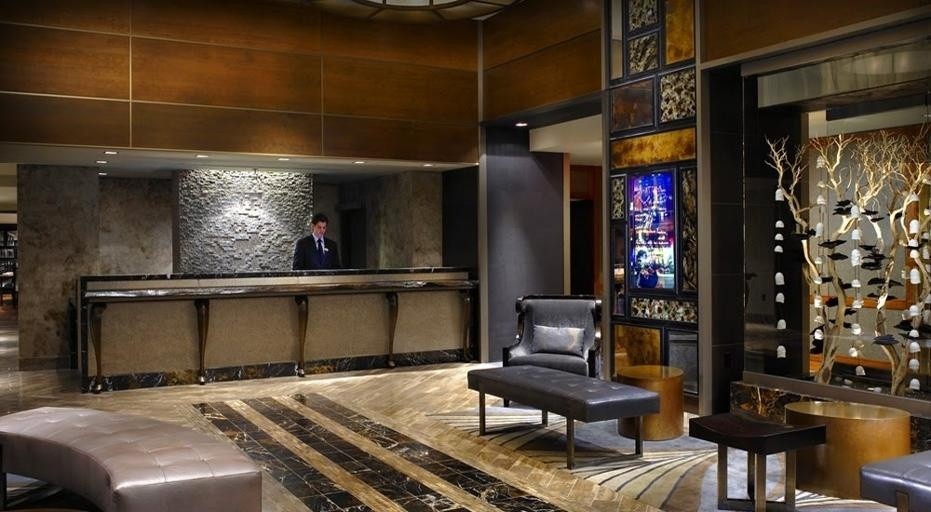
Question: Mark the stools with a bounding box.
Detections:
[617,365,684,441]
[689,409,825,512]
[784,401,911,500]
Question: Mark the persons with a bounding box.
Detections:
[293,211,345,275]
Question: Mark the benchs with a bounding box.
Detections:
[0,407,262,512]
[467,365,660,471]
[860,449,931,512]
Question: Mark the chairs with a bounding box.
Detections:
[502,294,603,407]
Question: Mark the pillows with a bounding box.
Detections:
[533,324,584,357]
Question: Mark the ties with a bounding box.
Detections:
[317,239,325,269]
